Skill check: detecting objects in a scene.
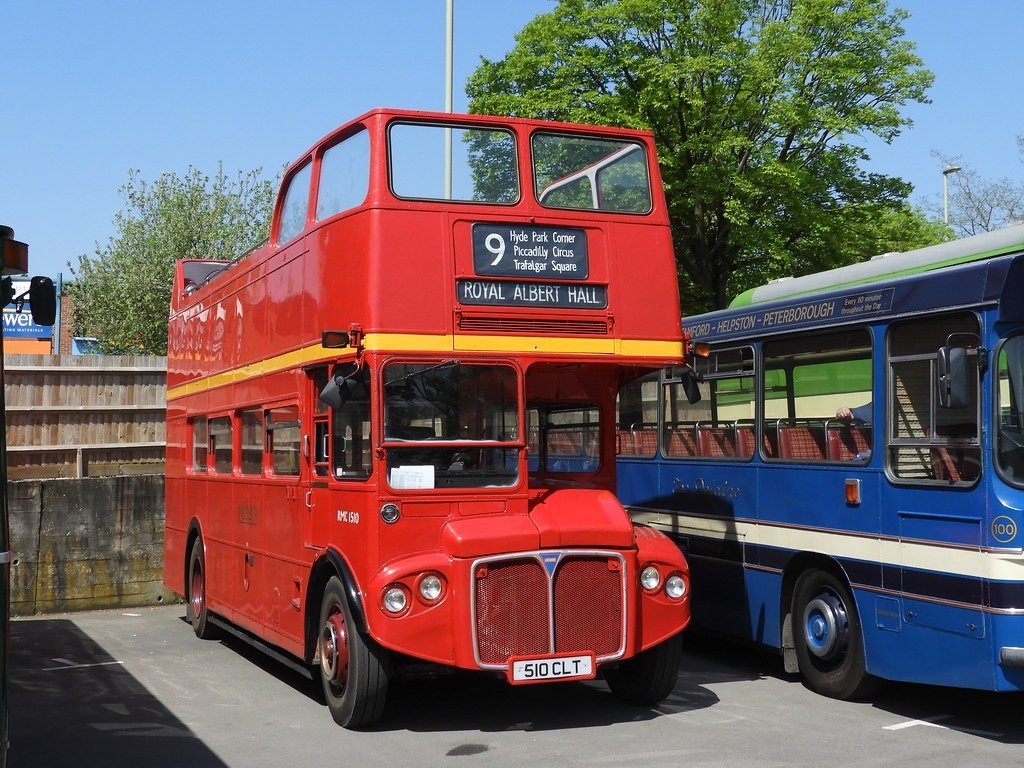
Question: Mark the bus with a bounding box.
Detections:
[489,218,1024,703]
[159,105,695,733]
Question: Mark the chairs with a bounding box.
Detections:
[926,421,981,481]
[192,416,873,474]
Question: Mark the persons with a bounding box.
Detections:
[836,401,873,460]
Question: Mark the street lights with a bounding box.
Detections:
[942,165,961,242]
